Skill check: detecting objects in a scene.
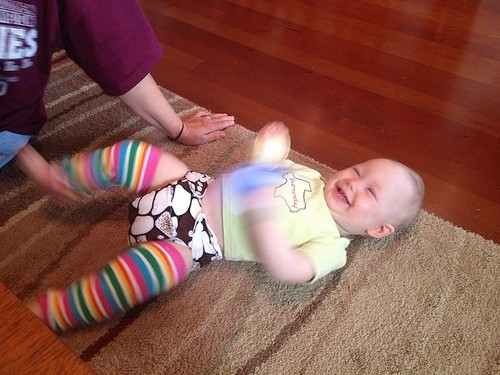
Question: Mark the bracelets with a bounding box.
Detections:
[166,123,184,142]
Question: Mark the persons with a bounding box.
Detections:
[18,122,425,333]
[0,0,236,168]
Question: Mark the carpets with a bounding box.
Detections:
[1,48,499,375]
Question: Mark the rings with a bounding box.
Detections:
[207,116,211,120]
